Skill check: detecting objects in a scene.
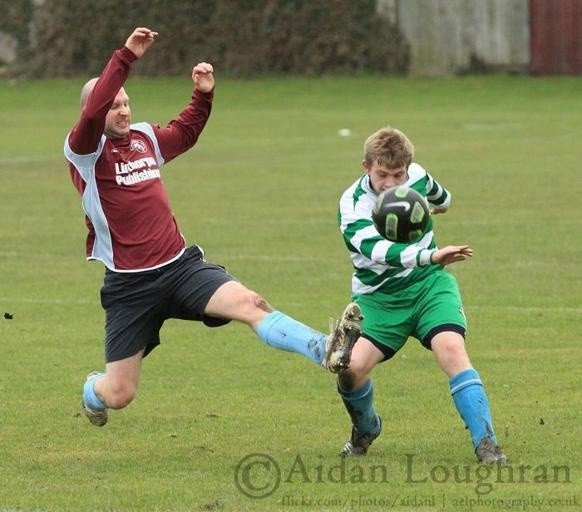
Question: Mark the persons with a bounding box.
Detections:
[62,26,362,428]
[332,126,510,465]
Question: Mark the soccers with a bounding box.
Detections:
[374,186,431,244]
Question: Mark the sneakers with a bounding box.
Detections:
[339,415,383,456]
[81,371,110,427]
[327,303,362,374]
[476,439,507,466]
